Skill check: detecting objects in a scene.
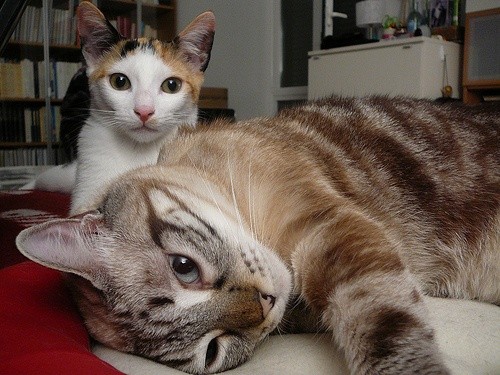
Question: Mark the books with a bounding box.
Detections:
[0,59,45,98]
[109,16,135,39]
[50,105,61,142]
[8,6,43,42]
[50,9,71,44]
[32,148,75,166]
[49,58,82,99]
[0,104,47,143]
[0,148,31,167]
[141,21,157,38]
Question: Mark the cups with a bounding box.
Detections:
[362,23,381,40]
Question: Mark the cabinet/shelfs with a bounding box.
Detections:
[0,0,179,146]
[307,36,463,101]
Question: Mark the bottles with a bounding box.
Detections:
[408,0,448,37]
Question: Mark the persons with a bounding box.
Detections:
[432,1,442,25]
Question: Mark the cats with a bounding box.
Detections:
[14,89,500,375]
[16,0,218,217]
